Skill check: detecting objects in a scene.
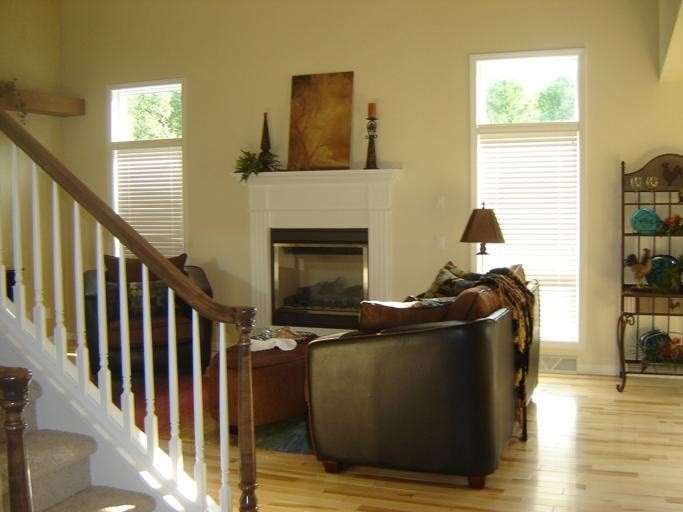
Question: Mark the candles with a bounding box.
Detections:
[367,103,376,118]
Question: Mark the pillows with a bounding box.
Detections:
[103,253,188,321]
[357,260,526,329]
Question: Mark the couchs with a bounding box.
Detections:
[303,277,541,488]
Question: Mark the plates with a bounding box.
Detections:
[645,256,681,294]
[631,208,660,235]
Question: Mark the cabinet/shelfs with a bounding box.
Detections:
[615,153,683,391]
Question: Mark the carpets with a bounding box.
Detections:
[112,365,314,455]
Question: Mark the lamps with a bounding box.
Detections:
[459,202,504,276]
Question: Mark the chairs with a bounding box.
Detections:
[82,265,213,376]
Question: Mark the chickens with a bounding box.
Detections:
[624,247,653,289]
